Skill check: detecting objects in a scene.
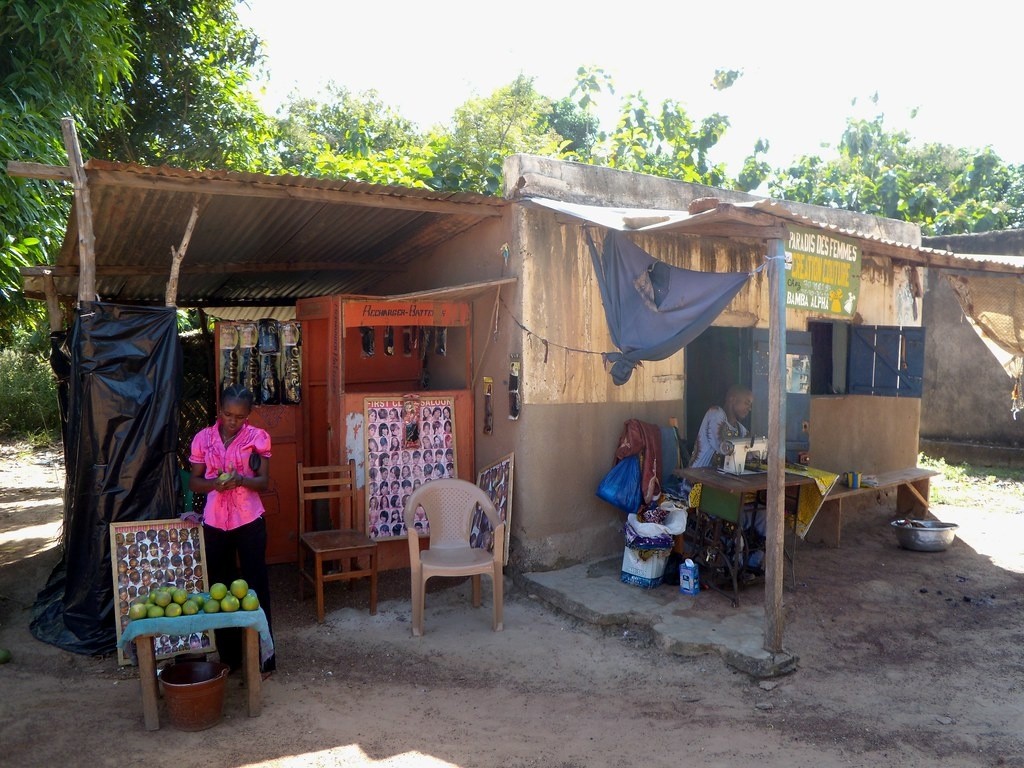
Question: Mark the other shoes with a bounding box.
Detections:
[724,565,755,581]
[239,670,270,687]
[746,566,766,579]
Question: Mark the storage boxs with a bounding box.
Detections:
[620,522,675,588]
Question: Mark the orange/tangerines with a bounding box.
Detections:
[129,579,260,620]
[219,473,231,481]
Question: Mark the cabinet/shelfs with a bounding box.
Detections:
[215,295,474,591]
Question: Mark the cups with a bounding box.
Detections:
[843,471,862,488]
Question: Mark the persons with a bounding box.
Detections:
[189,385,277,687]
[681,384,767,575]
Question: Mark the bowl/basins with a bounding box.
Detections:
[890,520,959,551]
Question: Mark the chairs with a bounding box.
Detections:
[296,459,377,623]
[403,479,504,637]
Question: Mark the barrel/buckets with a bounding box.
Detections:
[158,653,231,732]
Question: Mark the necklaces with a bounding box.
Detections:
[223,434,236,444]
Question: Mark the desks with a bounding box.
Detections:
[115,589,273,731]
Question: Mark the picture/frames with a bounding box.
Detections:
[364,396,458,542]
[468,451,515,565]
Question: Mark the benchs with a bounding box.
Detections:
[806,468,940,547]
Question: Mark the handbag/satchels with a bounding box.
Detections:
[594,452,641,513]
